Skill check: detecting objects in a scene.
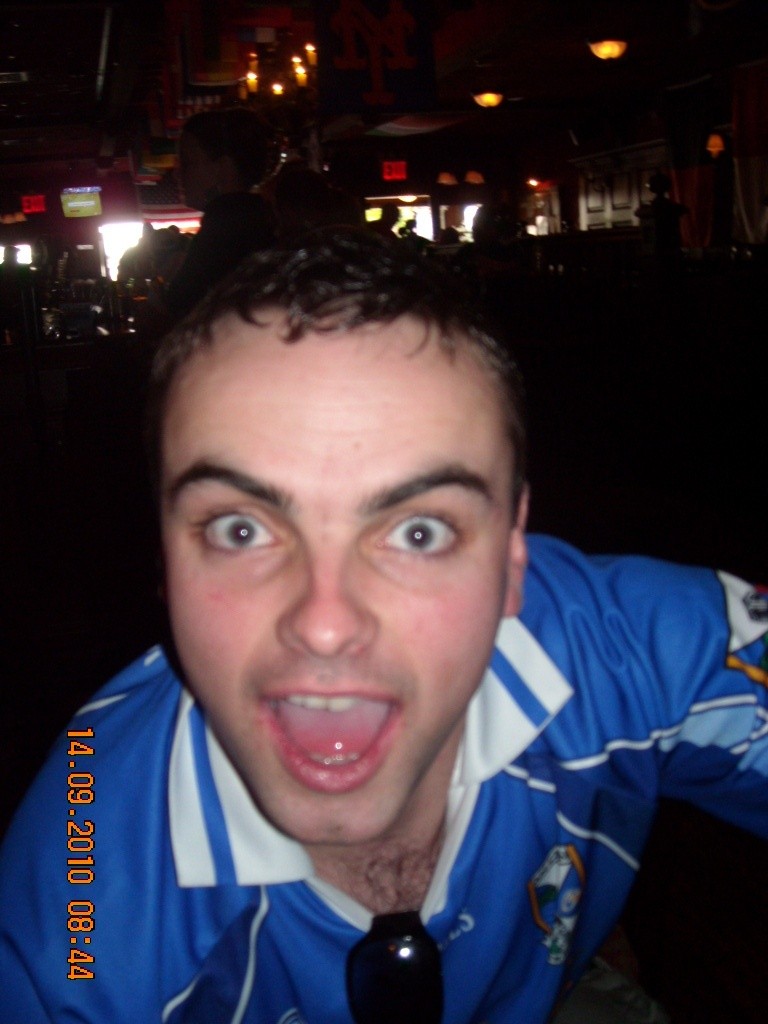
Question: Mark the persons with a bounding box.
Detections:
[0,222,768,1023]
[134,107,362,341]
[0,199,526,293]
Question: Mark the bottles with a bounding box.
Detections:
[104,276,166,333]
[177,95,222,119]
[40,282,63,334]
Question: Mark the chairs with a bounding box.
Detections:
[113,281,130,314]
[57,278,109,352]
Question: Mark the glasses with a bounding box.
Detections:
[343,913,445,1024]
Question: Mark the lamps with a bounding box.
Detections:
[469,87,504,110]
[584,29,629,61]
[382,152,407,180]
[435,171,458,185]
[464,170,485,185]
[98,170,145,234]
[705,133,726,159]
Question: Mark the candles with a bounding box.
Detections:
[243,42,317,95]
